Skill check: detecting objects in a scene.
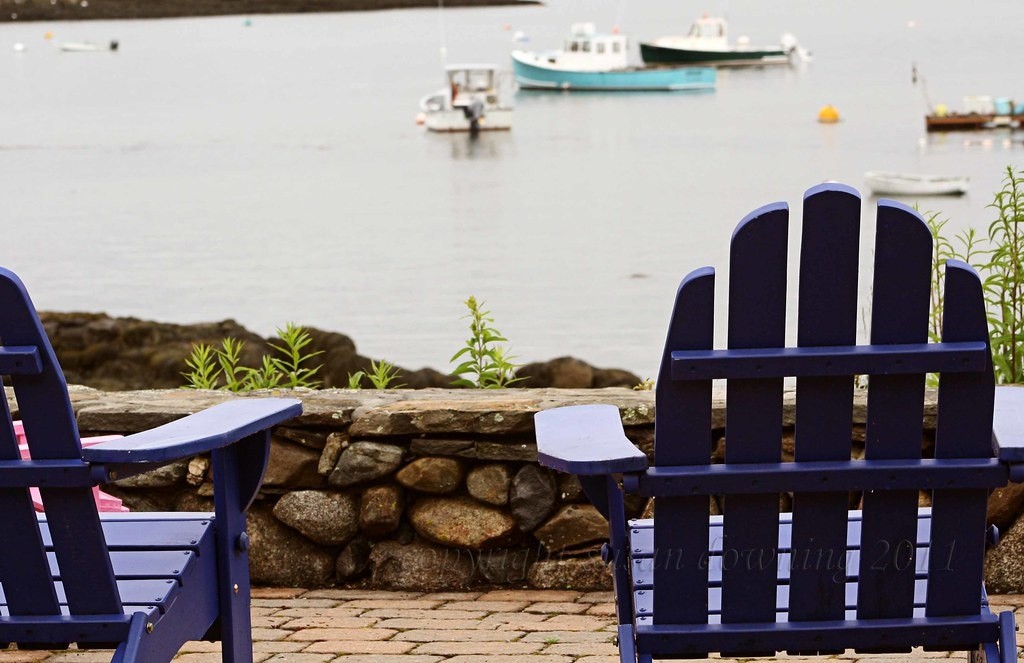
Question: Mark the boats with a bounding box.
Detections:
[924,101,1024,130]
[869,170,967,196]
[59,42,120,51]
[508,22,716,93]
[637,16,802,67]
[415,64,511,132]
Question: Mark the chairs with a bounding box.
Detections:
[0,184,305,663]
[533,182,1024,663]
